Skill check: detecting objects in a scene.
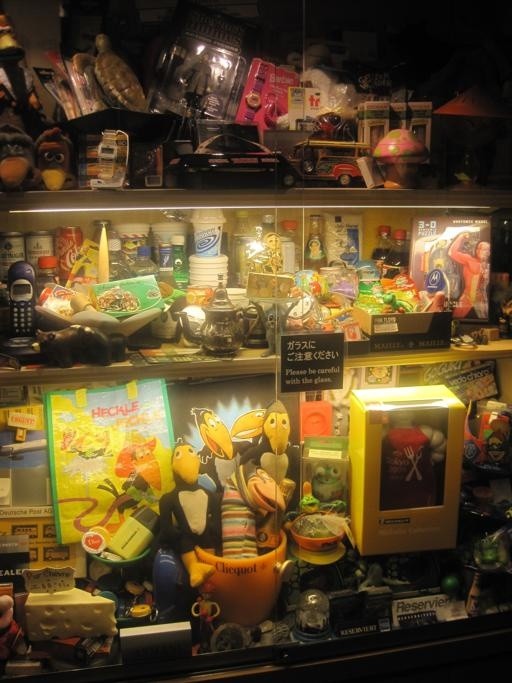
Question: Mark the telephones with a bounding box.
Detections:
[0,261,46,355]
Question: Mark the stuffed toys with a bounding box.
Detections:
[1,25,510,661]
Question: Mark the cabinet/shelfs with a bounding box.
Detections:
[0,178,510,683]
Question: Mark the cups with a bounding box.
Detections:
[111,207,226,270]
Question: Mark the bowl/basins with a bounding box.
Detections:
[290,512,345,550]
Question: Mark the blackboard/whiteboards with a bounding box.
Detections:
[276,328,348,396]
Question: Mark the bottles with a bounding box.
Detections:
[168,232,190,289]
[89,218,114,246]
[372,224,409,274]
[462,571,485,615]
[228,209,322,284]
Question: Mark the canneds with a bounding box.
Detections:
[0,231,25,283]
[25,230,54,270]
[55,224,83,286]
